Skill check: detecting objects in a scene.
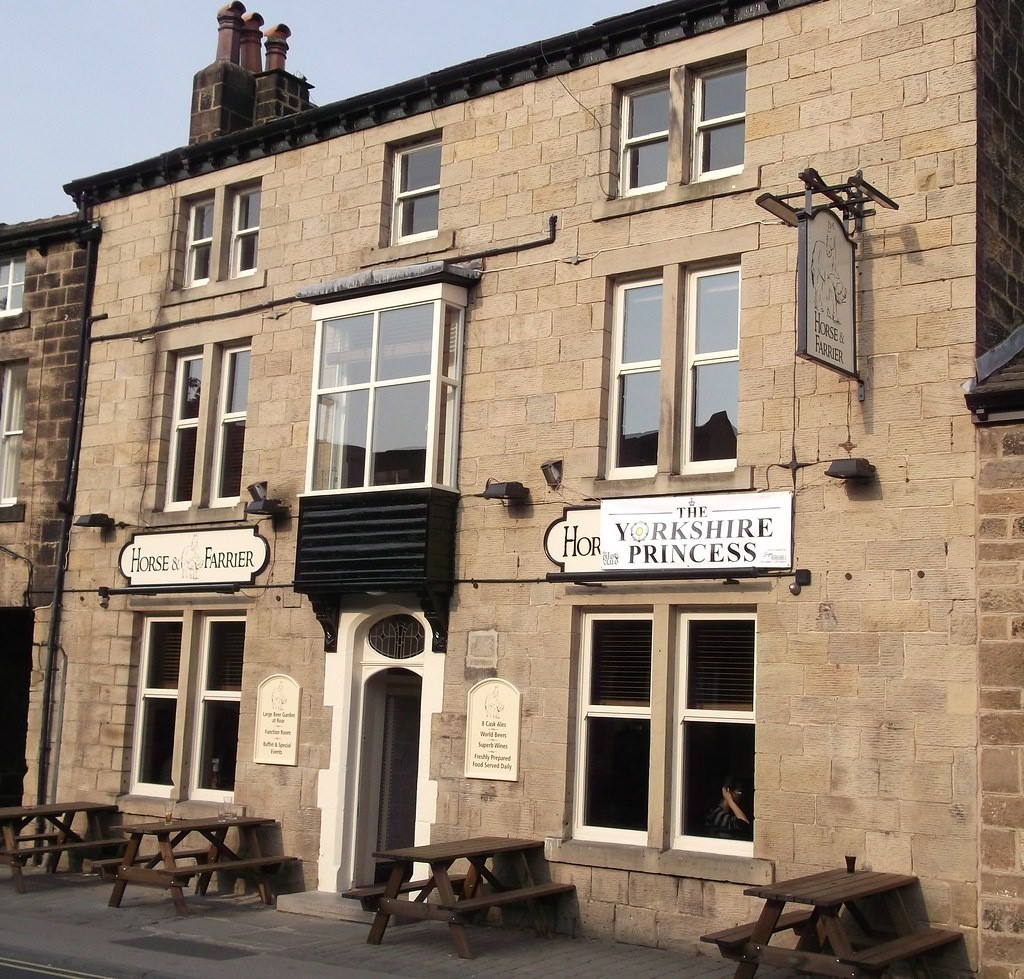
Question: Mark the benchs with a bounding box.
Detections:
[0,832,298,889]
[341,874,575,927]
[699,910,964,979]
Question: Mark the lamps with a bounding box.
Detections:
[846,176,900,211]
[474,479,530,499]
[824,457,876,480]
[755,192,804,228]
[244,498,292,516]
[72,513,130,529]
[963,388,995,413]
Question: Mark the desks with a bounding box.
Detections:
[106,816,276,917]
[0,801,119,893]
[366,835,555,960]
[734,867,921,979]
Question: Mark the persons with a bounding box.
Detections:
[705,777,750,838]
